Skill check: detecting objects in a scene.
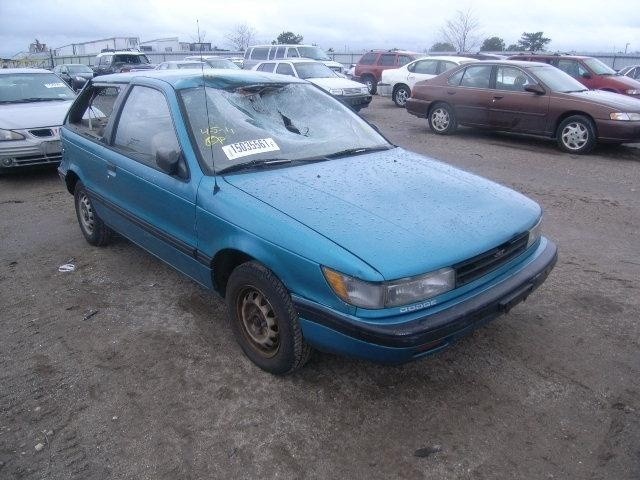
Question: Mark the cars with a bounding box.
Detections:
[0,67,106,172]
[349,45,640,153]
[57,66,557,379]
